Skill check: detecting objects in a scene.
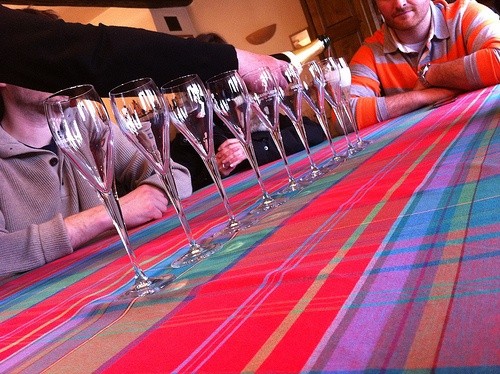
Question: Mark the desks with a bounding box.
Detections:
[0,83,500,374]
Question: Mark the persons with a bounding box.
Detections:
[0,82,193,280]
[166,30,326,190]
[331,0,500,134]
[0,4,295,98]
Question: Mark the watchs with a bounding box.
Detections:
[416,63,432,90]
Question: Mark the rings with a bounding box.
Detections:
[222,164,227,169]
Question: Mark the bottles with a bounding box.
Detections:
[270,35,331,75]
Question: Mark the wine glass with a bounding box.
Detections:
[43,83,176,302]
[330,57,373,154]
[205,70,289,215]
[310,57,362,160]
[161,74,260,237]
[294,60,351,169]
[240,65,315,195]
[108,76,224,269]
[269,66,330,187]
